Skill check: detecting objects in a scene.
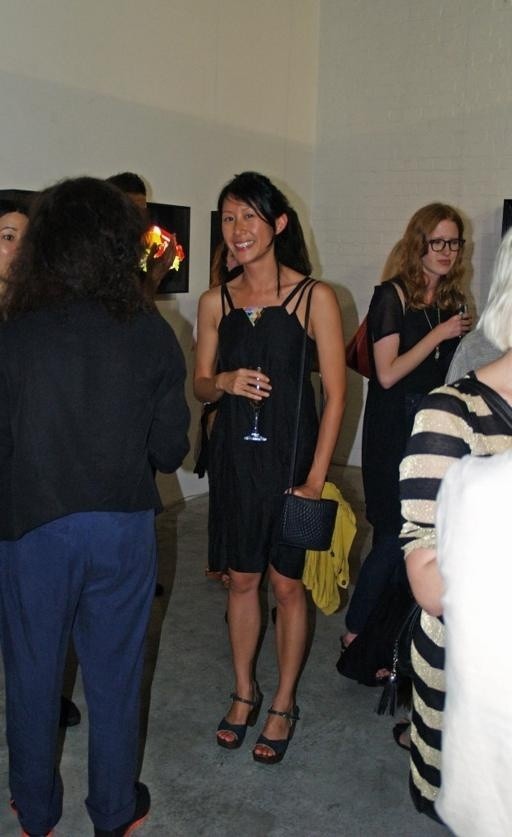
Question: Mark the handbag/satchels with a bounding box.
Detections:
[277,493,339,552]
[345,280,408,380]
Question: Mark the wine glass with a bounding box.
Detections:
[242,364,271,444]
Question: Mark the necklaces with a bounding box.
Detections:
[421,306,443,359]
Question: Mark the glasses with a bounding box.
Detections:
[425,239,466,251]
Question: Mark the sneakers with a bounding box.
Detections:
[60,695,82,727]
[93,782,151,837]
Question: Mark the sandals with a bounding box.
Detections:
[252,704,300,764]
[392,722,411,750]
[215,691,262,750]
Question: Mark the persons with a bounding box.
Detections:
[0,199,81,726]
[432,446,512,835]
[194,172,347,765]
[332,203,470,681]
[197,235,244,588]
[446,222,512,383]
[395,352,512,823]
[108,170,182,596]
[1,174,194,834]
